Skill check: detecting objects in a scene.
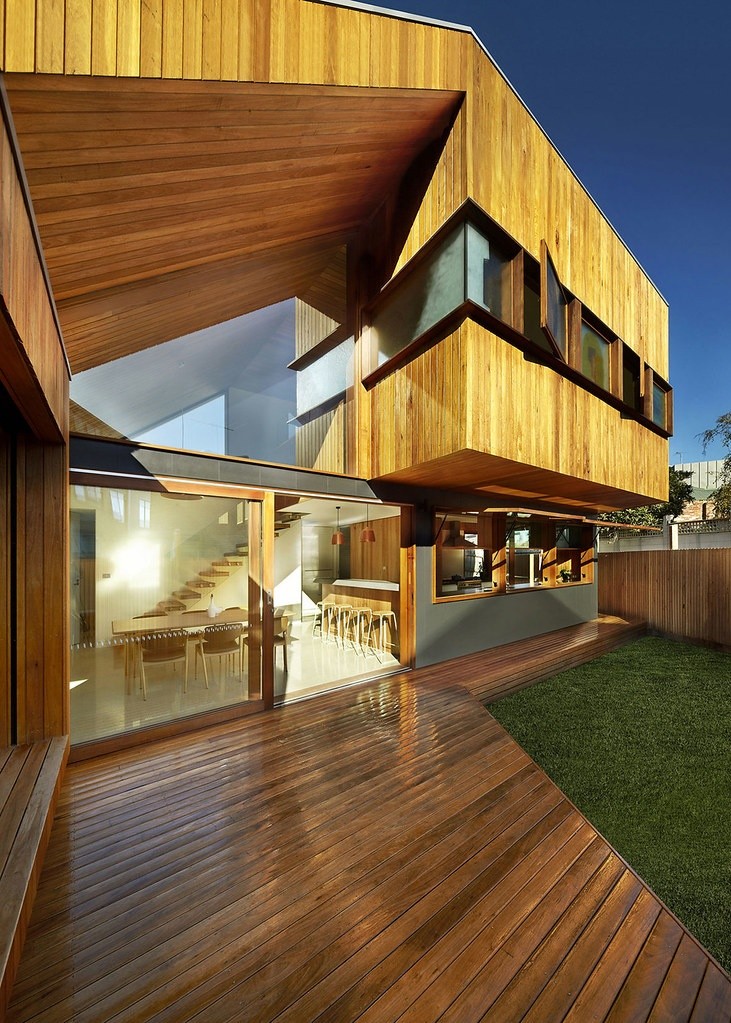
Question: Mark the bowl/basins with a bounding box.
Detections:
[206,607,223,616]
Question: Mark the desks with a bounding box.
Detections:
[112,607,296,696]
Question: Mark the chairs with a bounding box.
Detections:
[129,607,288,701]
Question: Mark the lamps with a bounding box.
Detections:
[331,507,345,545]
[359,504,376,542]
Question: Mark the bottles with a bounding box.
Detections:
[208,594,216,617]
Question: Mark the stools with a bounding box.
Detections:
[365,610,401,665]
[343,607,378,657]
[311,601,338,643]
[327,603,357,648]
